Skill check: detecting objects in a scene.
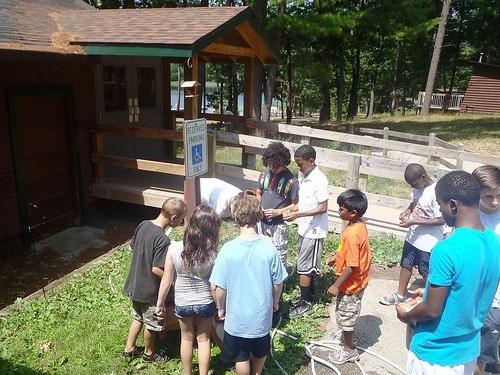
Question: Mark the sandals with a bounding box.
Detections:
[140,352,166,363]
[123,346,143,357]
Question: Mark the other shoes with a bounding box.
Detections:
[289,294,318,305]
[284,302,314,318]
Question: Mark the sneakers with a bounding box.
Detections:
[329,347,359,364]
[329,330,356,346]
[379,293,407,305]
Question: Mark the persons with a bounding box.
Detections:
[379,163,445,305]
[282,145,329,319]
[200,178,257,219]
[256,142,299,270]
[395,170,500,375]
[156,205,223,375]
[122,197,188,362]
[206,104,234,133]
[443,165,500,375]
[209,195,288,375]
[326,189,373,365]
